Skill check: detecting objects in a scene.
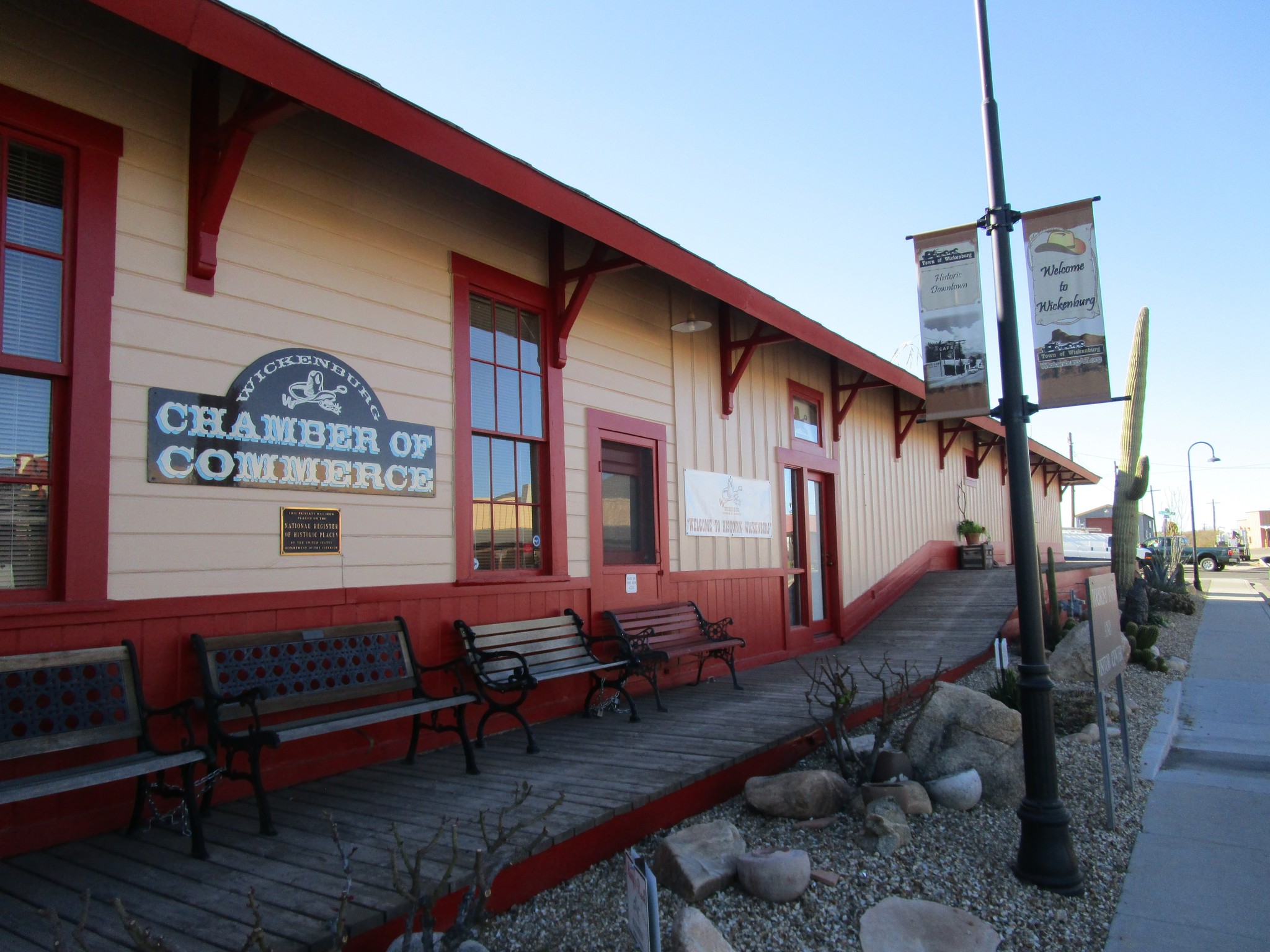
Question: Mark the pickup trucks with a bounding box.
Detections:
[1140,537,1240,571]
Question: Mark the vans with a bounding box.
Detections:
[1060,527,1153,565]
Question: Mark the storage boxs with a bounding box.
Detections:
[961,545,994,570]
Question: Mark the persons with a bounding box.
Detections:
[1148,539,1160,547]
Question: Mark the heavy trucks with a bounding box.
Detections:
[1216,527,1250,560]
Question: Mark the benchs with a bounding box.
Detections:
[191,614,483,834]
[0,640,213,863]
[603,604,747,713]
[455,608,641,754]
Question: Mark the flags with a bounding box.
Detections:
[1019,197,1112,410]
[910,221,991,422]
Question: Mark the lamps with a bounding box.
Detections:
[671,283,713,333]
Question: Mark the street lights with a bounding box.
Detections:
[1188,441,1220,591]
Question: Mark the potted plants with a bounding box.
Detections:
[957,519,989,545]
